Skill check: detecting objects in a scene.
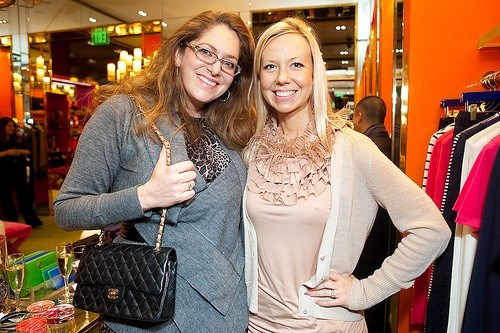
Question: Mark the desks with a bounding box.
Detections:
[0,284,101,333]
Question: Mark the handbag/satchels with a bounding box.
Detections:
[72,242,178,328]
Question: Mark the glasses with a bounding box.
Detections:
[183,40,242,77]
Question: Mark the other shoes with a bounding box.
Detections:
[22,213,44,226]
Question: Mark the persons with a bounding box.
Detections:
[0,118,42,228]
[238,17,452,333]
[52,11,256,333]
[350,93,399,333]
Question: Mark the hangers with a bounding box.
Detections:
[440,71,500,120]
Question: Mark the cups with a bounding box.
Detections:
[70,245,86,271]
[0,234,10,312]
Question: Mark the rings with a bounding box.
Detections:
[332,289,336,299]
[187,181,193,191]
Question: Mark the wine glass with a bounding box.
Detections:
[7,253,26,312]
[55,241,76,303]
